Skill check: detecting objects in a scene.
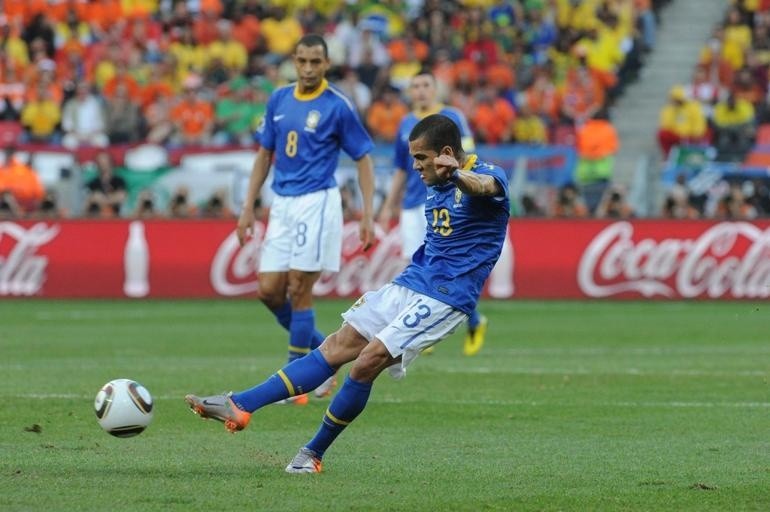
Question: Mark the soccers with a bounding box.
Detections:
[95,379,153,438]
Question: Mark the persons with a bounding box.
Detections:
[379,70,487,356]
[237,35,375,405]
[184,114,510,475]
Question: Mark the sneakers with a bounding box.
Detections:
[273,393,308,404]
[314,375,337,398]
[284,447,321,474]
[184,391,253,433]
[463,314,487,356]
